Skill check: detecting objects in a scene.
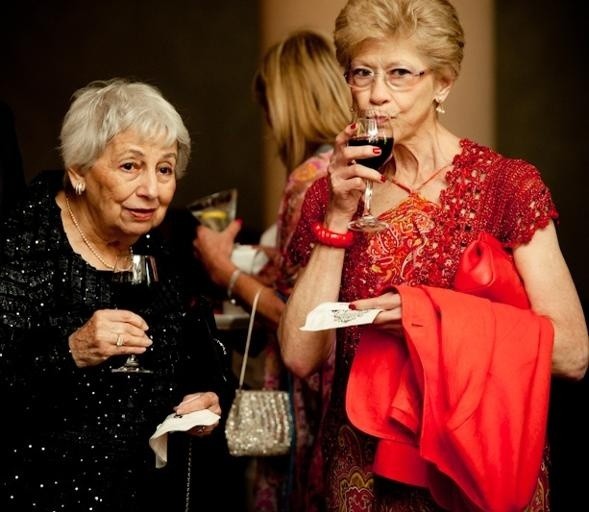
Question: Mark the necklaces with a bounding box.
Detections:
[62,195,136,272]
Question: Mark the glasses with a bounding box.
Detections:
[343,61,437,92]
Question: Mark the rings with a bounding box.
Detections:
[113,333,126,349]
[196,426,206,433]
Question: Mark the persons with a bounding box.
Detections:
[1,71,241,509]
[270,2,589,512]
[193,24,362,329]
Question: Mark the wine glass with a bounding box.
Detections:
[109,254,161,374]
[346,108,396,233]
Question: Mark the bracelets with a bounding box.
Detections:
[311,220,358,252]
[226,263,242,301]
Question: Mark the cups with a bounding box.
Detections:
[186,186,238,252]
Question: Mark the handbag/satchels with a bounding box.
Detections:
[225,389,297,459]
[454,232,531,312]
